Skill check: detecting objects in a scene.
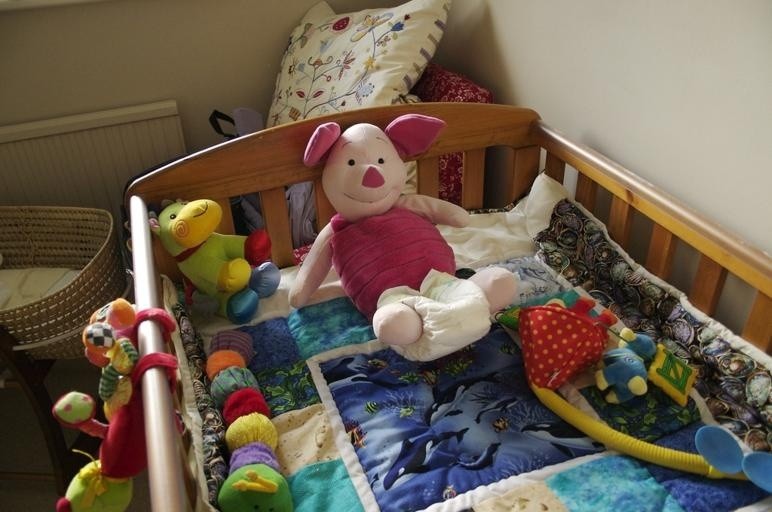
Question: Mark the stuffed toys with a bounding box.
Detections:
[495,293,619,392]
[286,112,520,363]
[148,197,283,326]
[206,329,294,512]
[592,326,658,406]
[50,297,183,512]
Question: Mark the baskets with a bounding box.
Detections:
[12,270,134,360]
[0,204,125,342]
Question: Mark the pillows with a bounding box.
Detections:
[266,0,451,128]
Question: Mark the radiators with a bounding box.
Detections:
[0,98,186,251]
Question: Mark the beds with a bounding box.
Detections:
[124,105,771,512]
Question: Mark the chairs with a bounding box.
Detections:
[296,62,494,266]
